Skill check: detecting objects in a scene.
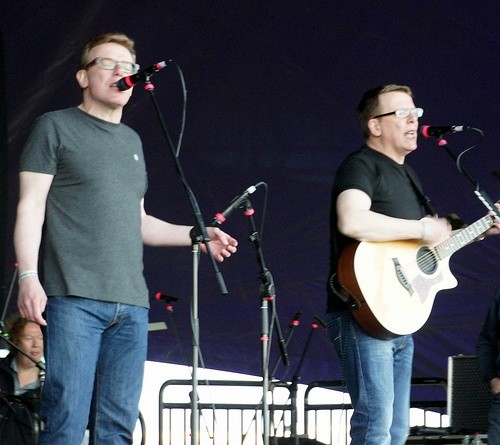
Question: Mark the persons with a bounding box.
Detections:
[328,84,500,445]
[15,33,238,445]
[0,317,46,420]
[477,286,500,445]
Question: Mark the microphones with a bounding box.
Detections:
[314,314,328,329]
[289,311,304,329]
[154,292,182,305]
[418,125,470,139]
[207,186,256,226]
[116,58,174,90]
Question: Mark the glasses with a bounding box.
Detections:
[83,58,140,74]
[374,108,424,118]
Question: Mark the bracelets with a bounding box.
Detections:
[17,270,38,280]
[474,237,485,242]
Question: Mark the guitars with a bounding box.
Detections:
[338,202,500,340]
